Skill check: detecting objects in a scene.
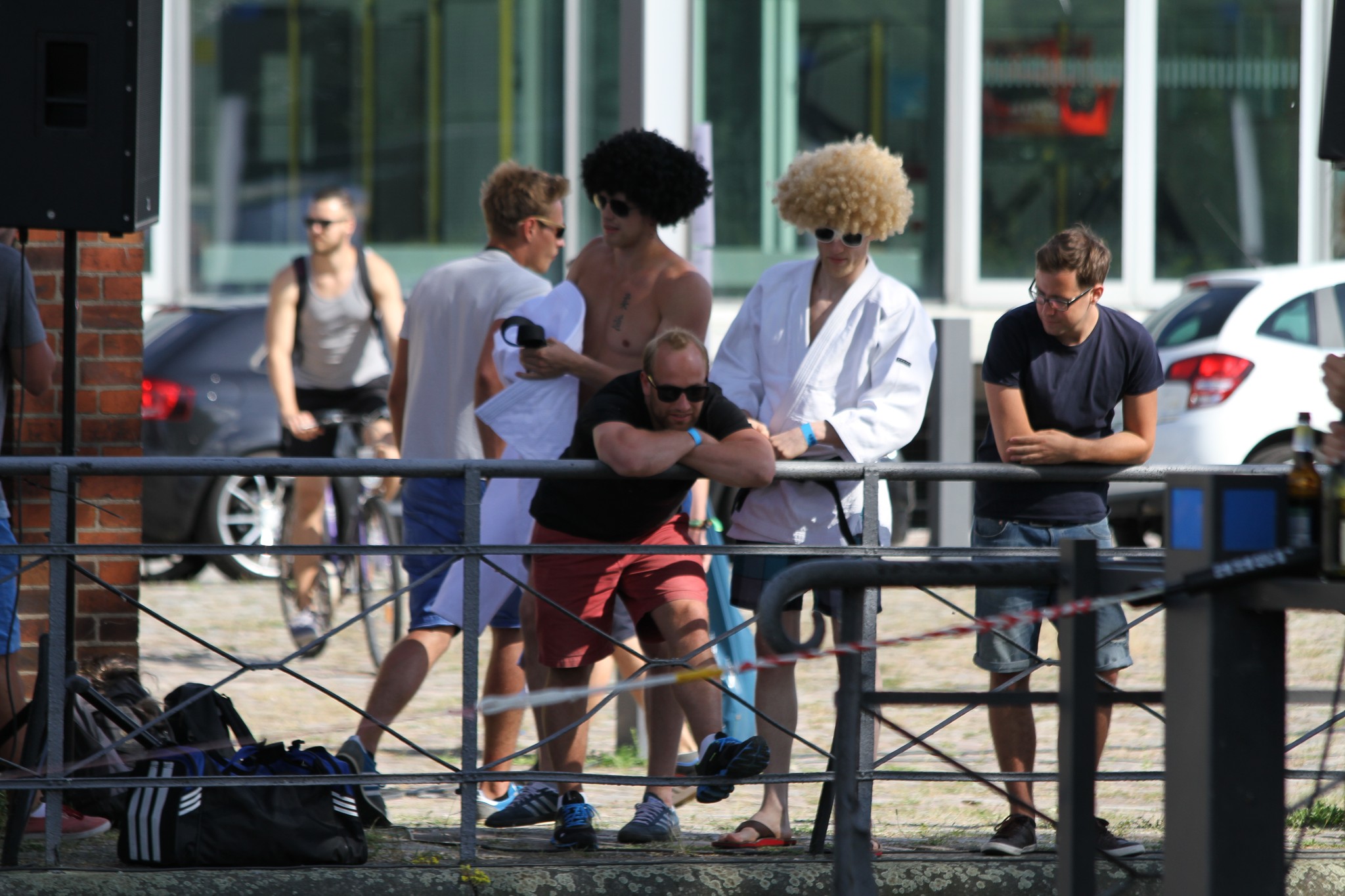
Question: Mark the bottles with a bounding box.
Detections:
[1323,469,1345,583]
[1282,412,1323,580]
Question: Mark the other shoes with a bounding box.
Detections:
[289,606,319,650]
[364,526,393,576]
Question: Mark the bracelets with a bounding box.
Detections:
[800,422,817,448]
[687,428,702,446]
[688,519,711,531]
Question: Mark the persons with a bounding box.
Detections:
[700,137,937,858]
[485,128,758,828]
[970,224,1165,858]
[0,227,110,840]
[1319,351,1345,467]
[530,328,776,852]
[333,159,572,827]
[264,189,411,654]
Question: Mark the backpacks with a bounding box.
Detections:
[19,682,128,773]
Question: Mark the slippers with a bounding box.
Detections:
[870,833,883,857]
[712,820,797,848]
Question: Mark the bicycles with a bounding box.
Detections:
[272,403,405,671]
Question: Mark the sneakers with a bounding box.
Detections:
[672,759,701,795]
[1094,817,1146,857]
[25,801,111,839]
[617,793,680,844]
[551,789,601,849]
[335,735,393,825]
[980,813,1037,856]
[483,778,586,828]
[695,731,771,804]
[476,781,525,823]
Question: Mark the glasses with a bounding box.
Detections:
[303,217,333,228]
[518,215,567,238]
[813,226,870,248]
[648,375,711,403]
[593,192,640,218]
[1028,279,1093,311]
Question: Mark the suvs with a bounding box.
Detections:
[142,294,418,582]
[1105,261,1344,585]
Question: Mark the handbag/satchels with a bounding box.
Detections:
[116,740,370,870]
[164,683,258,762]
